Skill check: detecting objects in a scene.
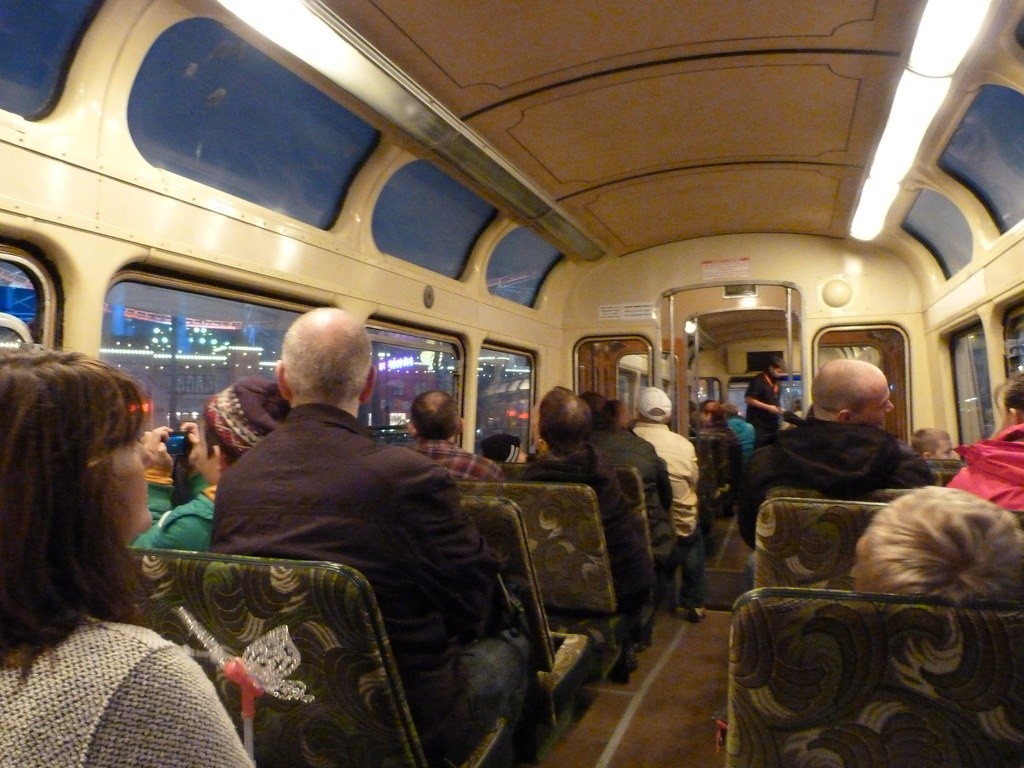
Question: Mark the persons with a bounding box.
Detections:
[116,366,295,560]
[627,389,711,624]
[702,399,720,410]
[695,405,742,503]
[712,481,1023,756]
[741,359,787,445]
[940,368,1023,513]
[717,404,757,490]
[202,304,533,768]
[389,387,511,487]
[0,342,266,768]
[908,427,964,465]
[688,399,699,438]
[733,357,938,551]
[576,391,676,549]
[780,393,804,429]
[525,385,654,681]
[480,432,529,463]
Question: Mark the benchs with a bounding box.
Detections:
[124,455,1024,768]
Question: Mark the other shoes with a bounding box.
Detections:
[690,606,705,621]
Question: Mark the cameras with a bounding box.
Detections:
[162,432,191,457]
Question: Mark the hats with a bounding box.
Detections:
[638,387,672,421]
[205,373,289,456]
[481,434,520,463]
